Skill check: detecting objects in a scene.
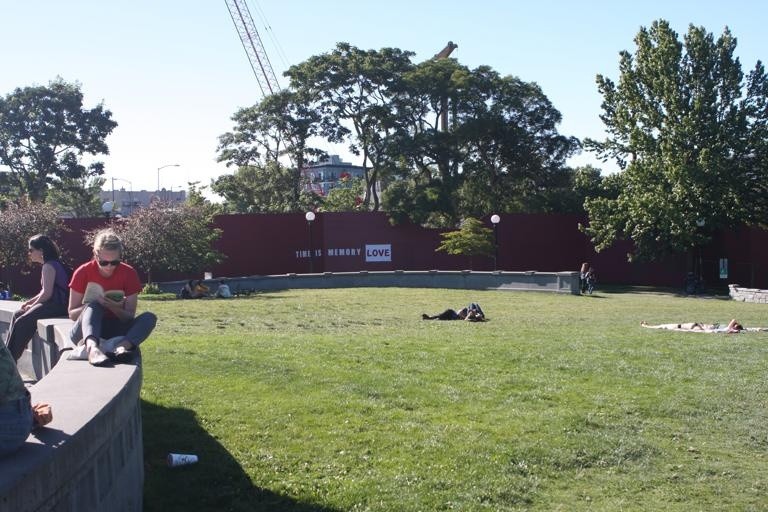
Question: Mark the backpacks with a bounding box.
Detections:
[55,260,74,293]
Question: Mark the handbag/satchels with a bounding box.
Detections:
[33,403,52,430]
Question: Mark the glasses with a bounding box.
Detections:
[98,260,120,267]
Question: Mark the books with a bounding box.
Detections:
[83,282,126,309]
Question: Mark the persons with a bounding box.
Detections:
[1,337,35,470]
[213,279,232,299]
[583,269,596,294]
[581,263,588,293]
[420,305,467,319]
[68,227,157,368]
[1,235,70,364]
[465,301,487,321]
[195,280,210,299]
[178,280,193,299]
[640,320,720,331]
[0,282,9,301]
[717,318,743,332]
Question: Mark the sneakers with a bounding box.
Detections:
[103,345,134,363]
[89,347,110,365]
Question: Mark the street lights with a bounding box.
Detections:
[111,177,129,207]
[304,210,317,274]
[490,214,501,270]
[170,184,183,202]
[157,163,180,201]
[102,201,114,229]
